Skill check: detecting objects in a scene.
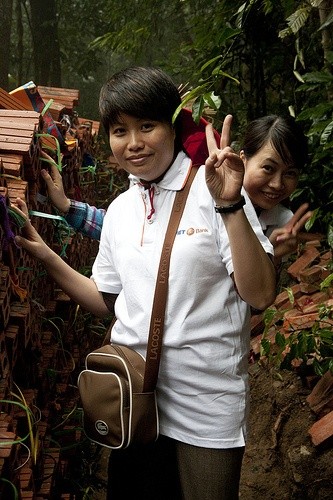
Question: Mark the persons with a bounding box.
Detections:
[36,114,314,259]
[9,62,278,500]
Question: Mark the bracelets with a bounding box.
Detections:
[214,194,245,214]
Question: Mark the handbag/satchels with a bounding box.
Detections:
[78,340,161,447]
